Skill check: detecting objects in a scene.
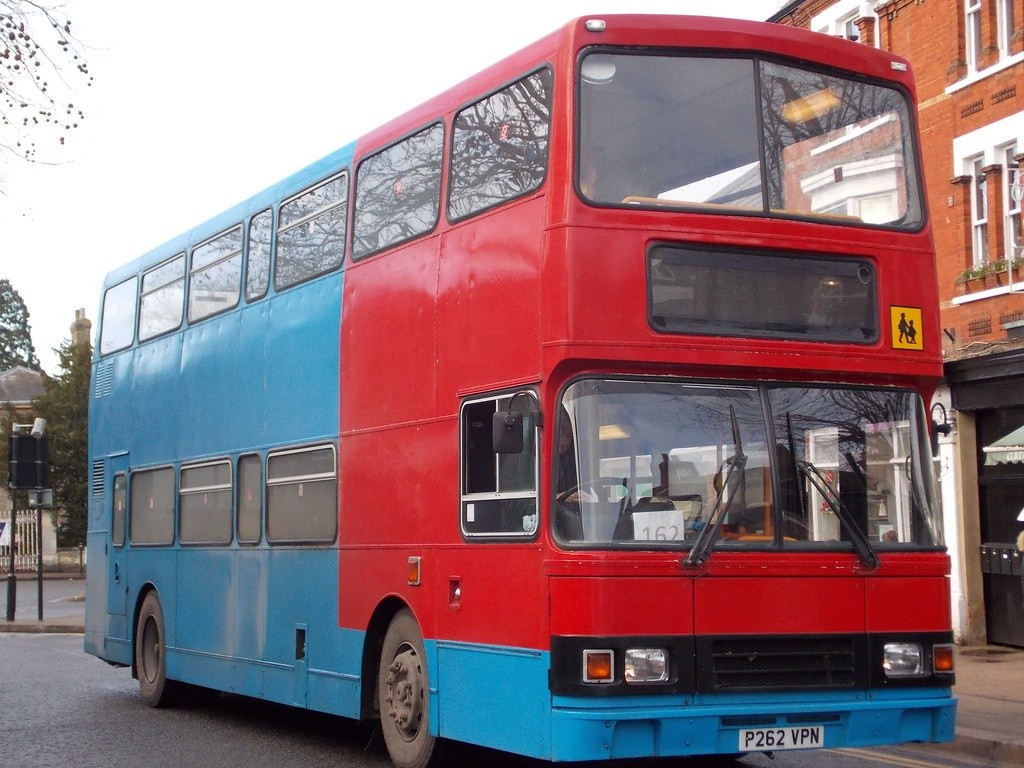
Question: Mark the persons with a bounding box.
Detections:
[500,410,598,531]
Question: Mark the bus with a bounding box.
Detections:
[83,14,959,768]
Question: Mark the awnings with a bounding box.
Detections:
[983,425,1024,466]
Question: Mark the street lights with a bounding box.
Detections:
[5,416,45,621]
